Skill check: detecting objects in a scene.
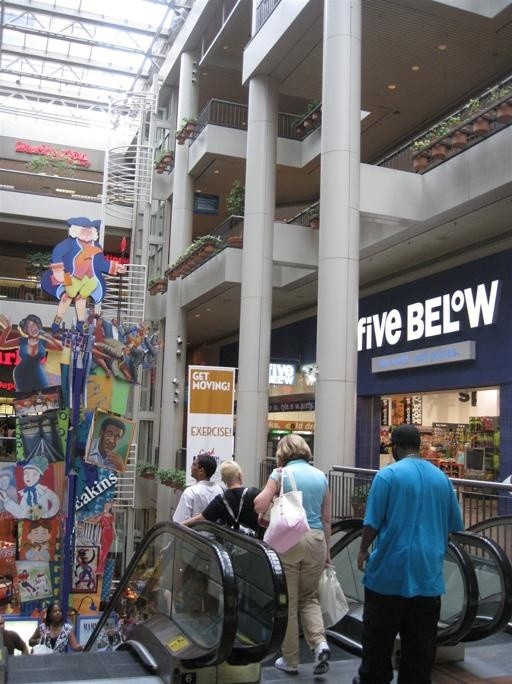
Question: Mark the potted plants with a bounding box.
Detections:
[412,81,512,169]
[147,237,219,295]
[291,101,322,134]
[176,117,195,144]
[138,463,186,490]
[155,150,173,172]
[307,206,319,229]
[227,180,244,248]
[351,483,371,518]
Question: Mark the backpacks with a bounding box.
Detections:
[221,488,253,537]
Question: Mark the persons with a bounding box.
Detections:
[0,616,28,655]
[1,455,62,521]
[159,453,226,612]
[25,520,53,561]
[87,417,125,470]
[252,433,335,676]
[29,603,86,652]
[0,313,64,393]
[180,460,267,611]
[352,425,464,684]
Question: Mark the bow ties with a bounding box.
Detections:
[24,483,40,507]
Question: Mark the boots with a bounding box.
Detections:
[77,319,88,337]
[50,314,62,337]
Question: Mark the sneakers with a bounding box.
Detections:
[311,642,330,676]
[273,655,300,676]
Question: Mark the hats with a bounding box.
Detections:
[23,455,50,477]
[65,216,102,230]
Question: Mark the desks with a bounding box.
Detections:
[380,454,395,469]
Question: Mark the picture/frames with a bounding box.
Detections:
[70,546,99,593]
[84,407,136,473]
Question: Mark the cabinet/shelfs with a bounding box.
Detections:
[438,463,463,487]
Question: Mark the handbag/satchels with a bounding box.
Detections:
[31,633,54,655]
[263,466,309,552]
[318,564,350,630]
